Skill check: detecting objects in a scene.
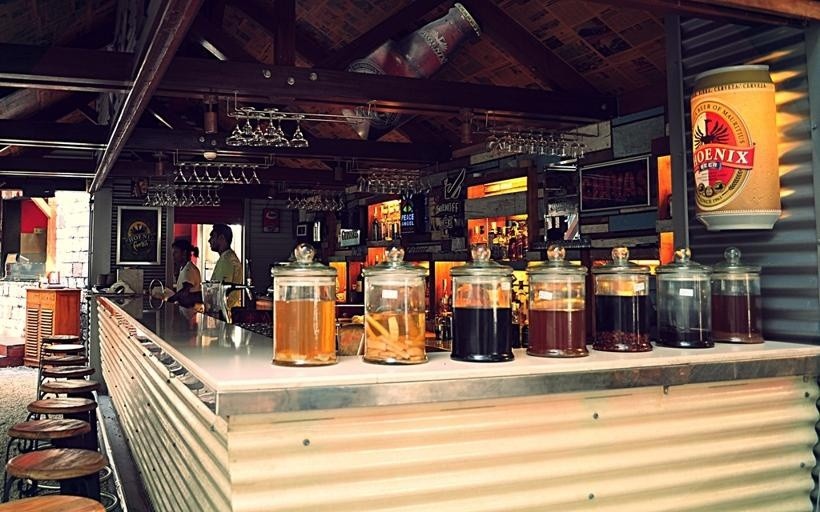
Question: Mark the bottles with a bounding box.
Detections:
[372,203,399,241]
[356,254,386,299]
[470,222,526,261]
[330,2,483,144]
[433,278,455,341]
[510,279,528,348]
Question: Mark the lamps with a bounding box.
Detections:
[202,94,218,132]
[459,108,474,144]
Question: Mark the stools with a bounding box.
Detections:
[1,329,118,512]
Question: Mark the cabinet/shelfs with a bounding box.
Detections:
[21,284,82,370]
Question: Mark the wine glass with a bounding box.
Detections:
[172,161,262,186]
[141,183,222,207]
[287,189,345,212]
[227,107,308,149]
[357,166,431,196]
[485,125,588,159]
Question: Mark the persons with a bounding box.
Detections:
[206,222,247,310]
[167,239,204,300]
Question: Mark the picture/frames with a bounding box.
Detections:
[113,204,164,266]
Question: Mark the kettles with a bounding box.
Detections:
[201,313,252,353]
[200,279,253,320]
